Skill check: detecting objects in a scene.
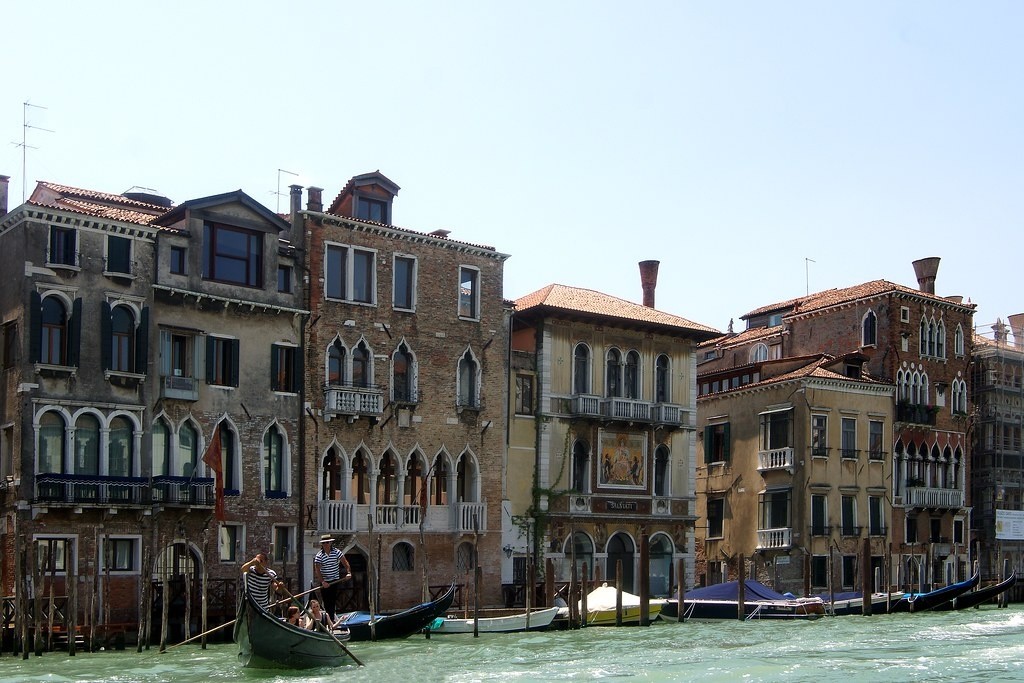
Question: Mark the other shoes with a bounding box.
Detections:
[332,621,336,624]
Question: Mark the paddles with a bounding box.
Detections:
[254,560,365,668]
[135,575,351,662]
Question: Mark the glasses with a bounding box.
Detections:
[322,542,329,544]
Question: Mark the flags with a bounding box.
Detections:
[202,425,225,524]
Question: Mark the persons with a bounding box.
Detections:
[676,524,684,546]
[315,535,351,622]
[552,522,564,547]
[596,524,606,551]
[283,606,304,628]
[241,554,282,612]
[306,600,348,631]
[636,524,647,541]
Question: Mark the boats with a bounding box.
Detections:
[897,569,979,612]
[578,582,669,626]
[931,570,1017,611]
[232,586,352,670]
[824,591,906,615]
[337,581,456,642]
[660,579,826,622]
[421,606,560,632]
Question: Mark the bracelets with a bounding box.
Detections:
[322,581,324,583]
[347,572,350,574]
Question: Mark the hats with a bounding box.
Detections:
[319,534,335,542]
